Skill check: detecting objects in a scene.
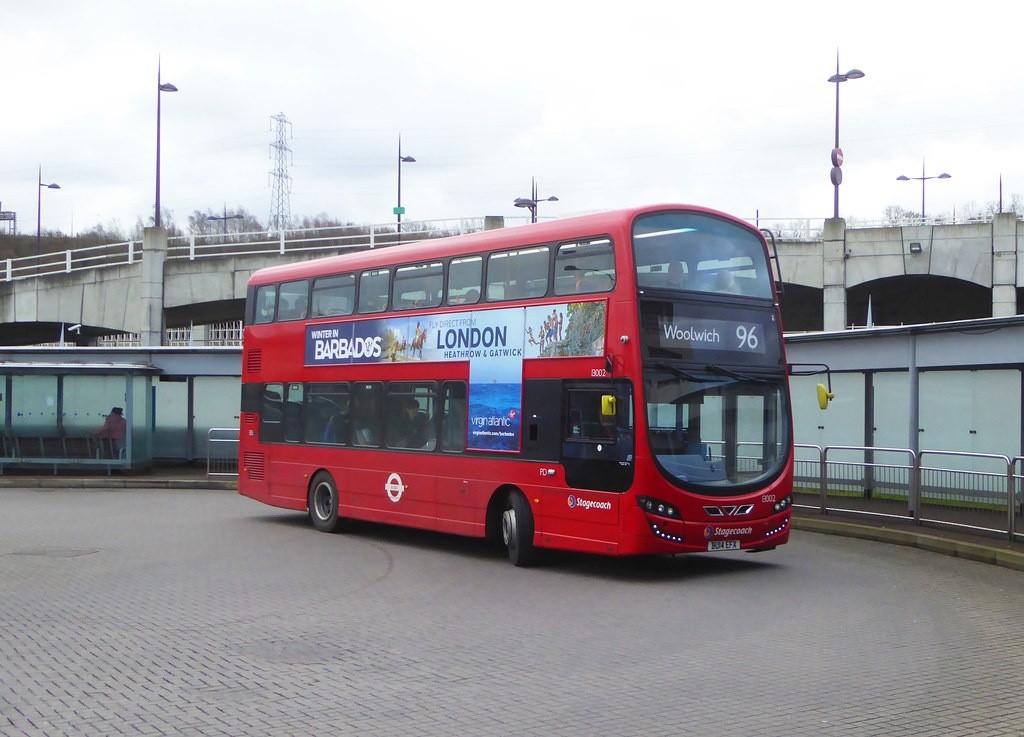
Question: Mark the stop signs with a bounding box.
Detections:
[831,148,843,167]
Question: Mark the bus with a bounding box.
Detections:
[236,199,837,568]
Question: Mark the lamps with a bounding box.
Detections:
[910,243,922,253]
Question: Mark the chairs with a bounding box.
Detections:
[577,278,600,291]
[415,299,434,307]
[322,414,336,443]
[326,308,347,315]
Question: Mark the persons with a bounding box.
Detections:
[392,399,430,448]
[664,260,686,289]
[94,407,126,459]
[416,321,422,345]
[464,289,480,304]
[525,309,562,354]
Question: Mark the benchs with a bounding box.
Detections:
[0,435,120,476]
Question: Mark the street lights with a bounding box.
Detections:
[207,202,245,245]
[514,174,560,223]
[35,164,62,274]
[150,53,178,228]
[895,155,953,225]
[827,46,867,219]
[395,132,418,245]
